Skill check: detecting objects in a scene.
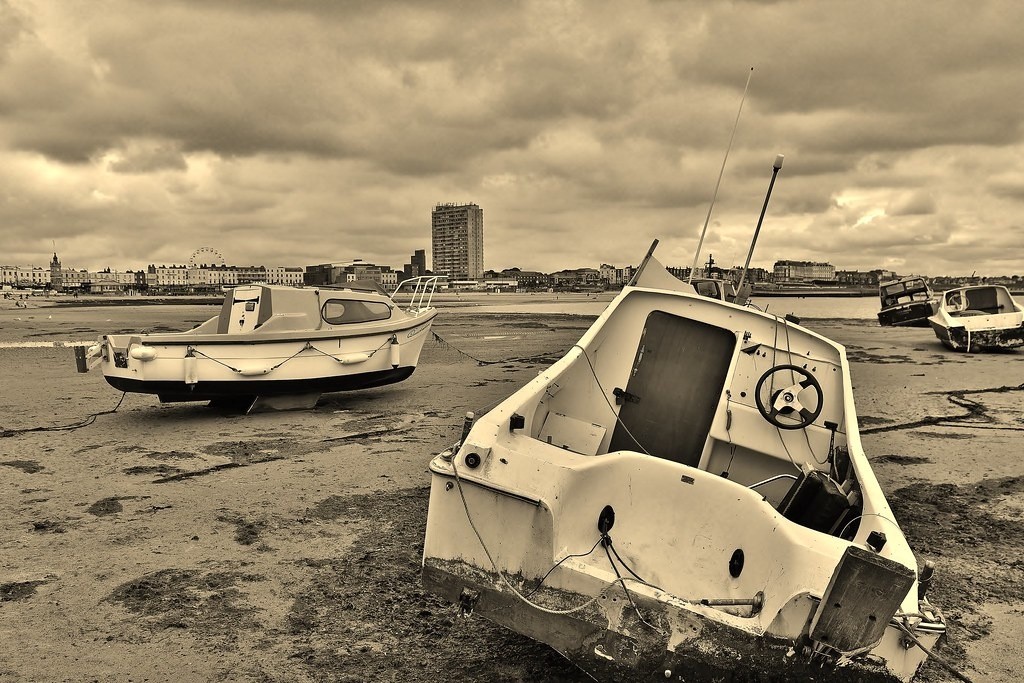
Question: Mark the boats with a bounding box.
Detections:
[875,270,940,329]
[414,151,950,683]
[75,273,452,414]
[928,283,1024,350]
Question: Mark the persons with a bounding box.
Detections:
[557,296,558,299]
[4,291,36,300]
[197,292,226,297]
[16,300,27,308]
[73,292,78,298]
[588,293,589,297]
[147,292,155,296]
